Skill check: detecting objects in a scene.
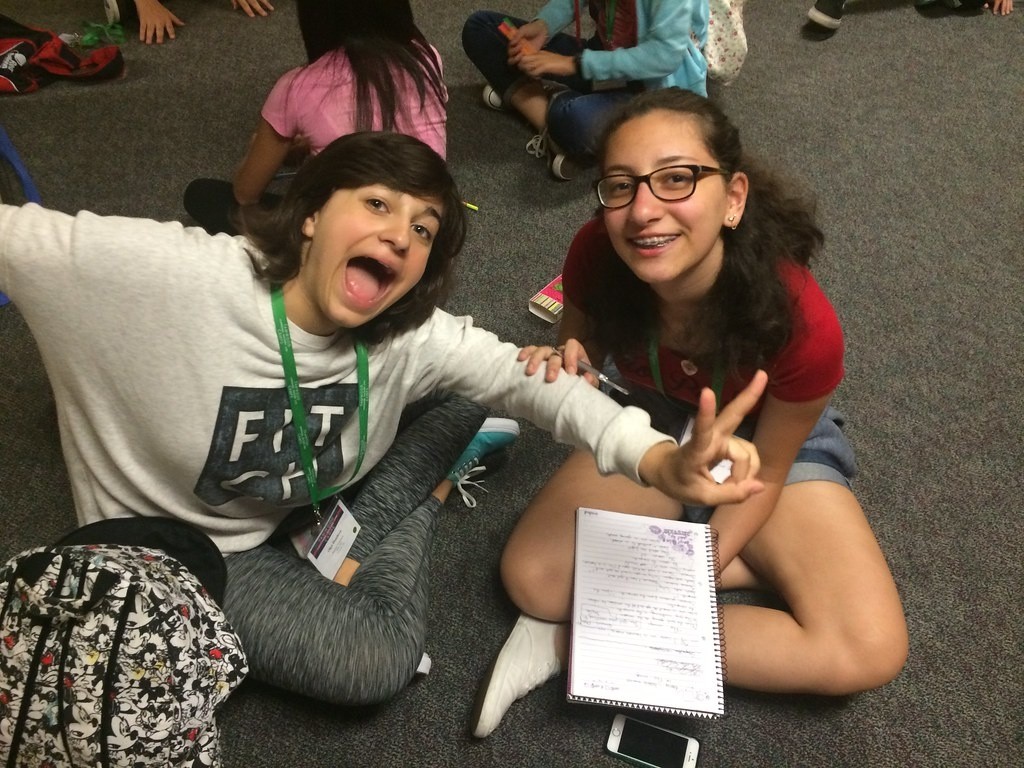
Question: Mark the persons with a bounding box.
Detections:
[808,0,1014,30]
[464,0,710,178]
[471,86,910,741]
[183,0,451,235]
[1,130,769,706]
[103,0,275,44]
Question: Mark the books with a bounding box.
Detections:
[566,506,731,720]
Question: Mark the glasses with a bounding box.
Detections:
[590,165,732,210]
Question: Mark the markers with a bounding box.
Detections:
[498,17,534,57]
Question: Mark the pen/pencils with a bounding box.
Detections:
[462,200,479,211]
[552,346,630,396]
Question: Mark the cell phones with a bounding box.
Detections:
[604,712,701,768]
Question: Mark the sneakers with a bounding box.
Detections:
[416,651,432,674]
[469,611,562,738]
[445,416,520,508]
[524,127,586,181]
[481,80,571,112]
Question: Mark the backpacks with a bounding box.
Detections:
[0,517,251,768]
[687,0,747,88]
[0,13,118,93]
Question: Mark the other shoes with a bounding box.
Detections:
[945,0,964,8]
[807,0,846,29]
